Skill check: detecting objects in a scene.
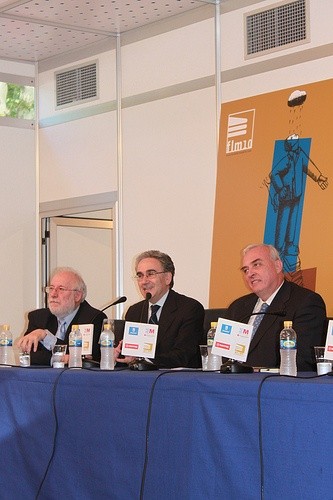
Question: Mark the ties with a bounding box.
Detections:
[58,321,66,339]
[251,304,267,339]
[149,305,161,324]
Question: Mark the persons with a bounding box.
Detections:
[220,242,327,372]
[17,267,108,367]
[113,251,205,370]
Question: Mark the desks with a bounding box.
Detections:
[1,364,333,499]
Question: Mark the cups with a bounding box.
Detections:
[199,344,209,371]
[313,346,332,376]
[19,347,30,368]
[50,344,67,369]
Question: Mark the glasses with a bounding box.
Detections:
[45,287,78,293]
[134,271,168,282]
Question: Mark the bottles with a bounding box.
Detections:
[67,325,82,369]
[206,322,224,374]
[279,321,297,377]
[0,324,17,367]
[99,324,114,371]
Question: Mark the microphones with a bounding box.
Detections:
[238,310,288,323]
[88,296,127,324]
[139,292,153,323]
